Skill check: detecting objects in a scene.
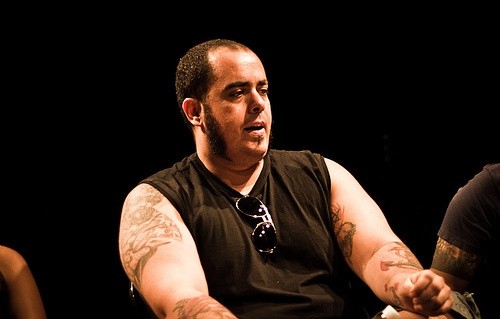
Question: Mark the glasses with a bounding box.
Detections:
[235,193,279,254]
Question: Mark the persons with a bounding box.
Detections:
[396,158,500,318]
[116,36,455,319]
